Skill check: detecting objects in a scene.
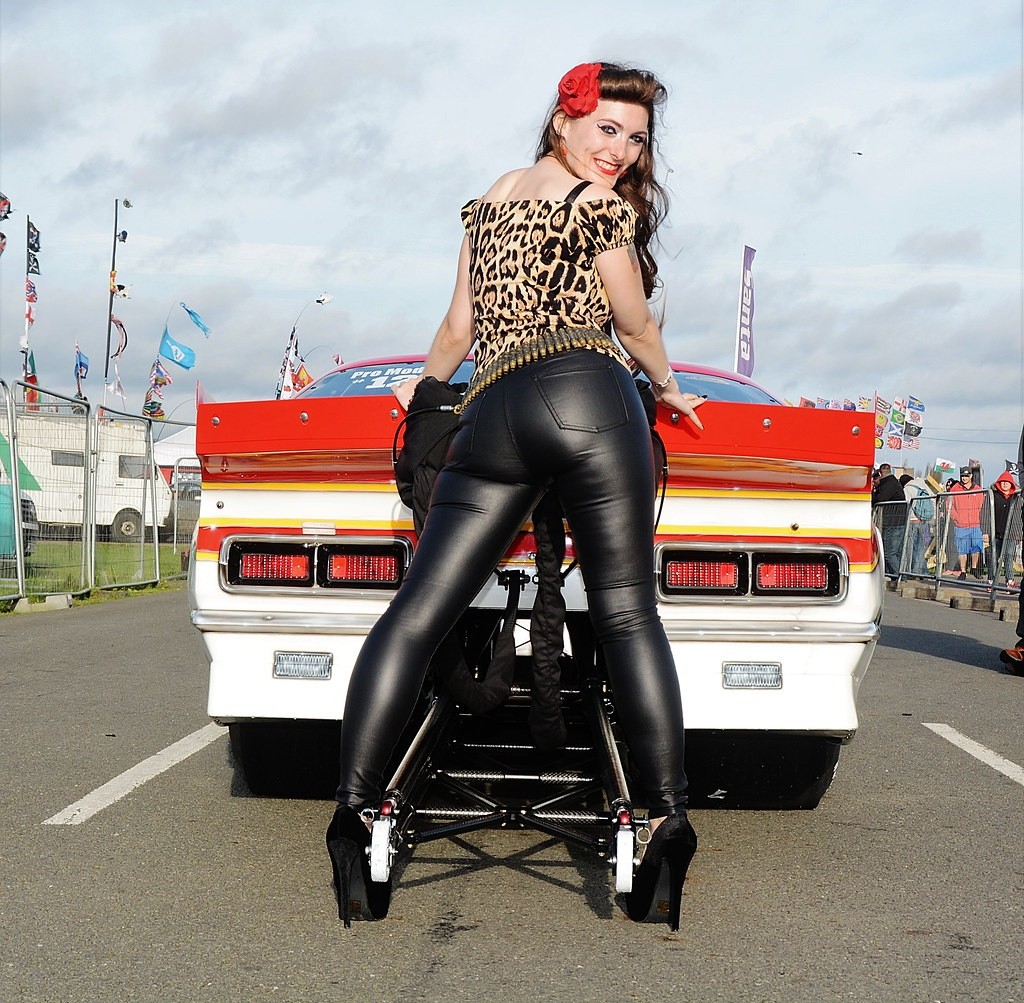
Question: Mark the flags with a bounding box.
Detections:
[74,350,89,380]
[142,325,196,420]
[26,221,40,326]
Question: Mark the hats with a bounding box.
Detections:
[960,466,972,476]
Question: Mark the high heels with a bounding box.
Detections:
[627,811,697,931]
[325,805,391,929]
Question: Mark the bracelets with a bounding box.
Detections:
[655,365,673,387]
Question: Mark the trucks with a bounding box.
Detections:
[0,413,182,546]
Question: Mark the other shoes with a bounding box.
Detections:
[1006,579,1017,594]
[970,566,983,579]
[987,579,997,593]
[999,647,1024,674]
[956,574,966,581]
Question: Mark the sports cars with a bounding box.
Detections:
[187,350,893,895]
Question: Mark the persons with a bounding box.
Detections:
[325,62,709,931]
[872,464,1022,594]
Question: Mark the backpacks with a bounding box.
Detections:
[906,484,935,522]
